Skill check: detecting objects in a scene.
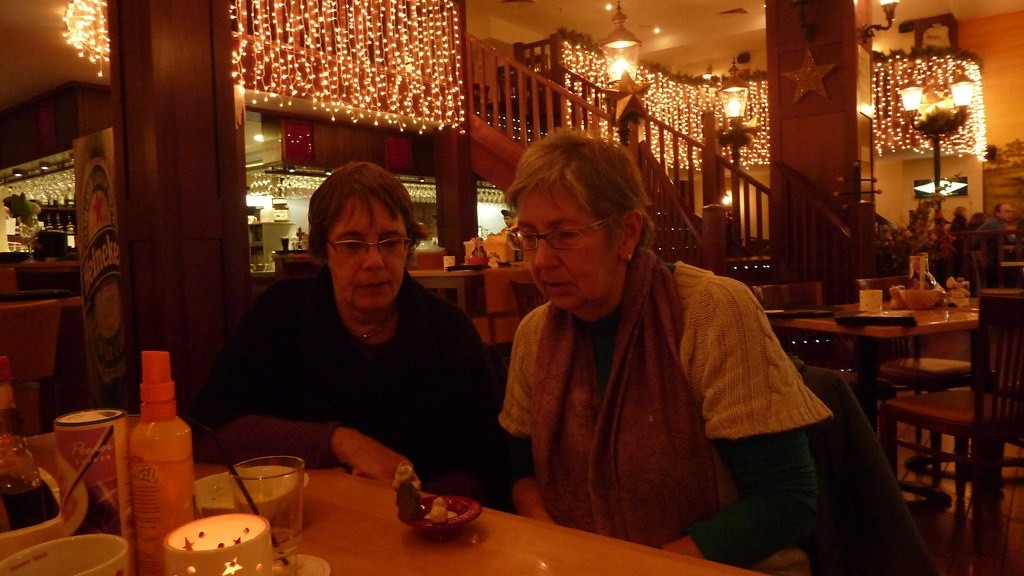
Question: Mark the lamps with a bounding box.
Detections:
[863,0,900,41]
[949,71,974,116]
[898,77,925,131]
[717,58,749,118]
[600,0,642,88]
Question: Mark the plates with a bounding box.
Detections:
[396,496,483,534]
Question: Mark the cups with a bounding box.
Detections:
[247,215,257,224]
[0,533,128,575]
[281,236,308,250]
[228,454,306,576]
[859,289,882,312]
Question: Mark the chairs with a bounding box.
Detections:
[502,280,548,321]
[473,343,535,515]
[717,229,1024,576]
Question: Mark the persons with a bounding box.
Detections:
[185,161,502,511]
[500,209,518,236]
[494,130,835,565]
[905,203,1014,265]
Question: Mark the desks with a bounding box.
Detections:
[407,266,486,326]
[766,305,978,506]
[178,462,762,576]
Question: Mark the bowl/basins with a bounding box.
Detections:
[467,256,488,265]
[194,466,310,524]
[900,289,939,308]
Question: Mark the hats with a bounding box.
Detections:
[501,210,511,215]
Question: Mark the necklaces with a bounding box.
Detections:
[344,307,394,343]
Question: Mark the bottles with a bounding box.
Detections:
[45,213,53,229]
[128,350,195,576]
[472,238,487,257]
[54,212,64,229]
[920,252,936,290]
[66,215,76,248]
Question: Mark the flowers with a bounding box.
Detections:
[878,173,960,253]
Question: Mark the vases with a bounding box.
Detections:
[912,248,936,288]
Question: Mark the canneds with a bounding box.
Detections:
[54,410,137,576]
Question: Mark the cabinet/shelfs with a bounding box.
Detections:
[245,221,296,268]
[38,204,78,235]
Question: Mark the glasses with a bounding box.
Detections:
[507,215,617,251]
[328,238,412,259]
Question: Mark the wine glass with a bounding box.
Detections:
[21,166,75,200]
[401,182,436,204]
[247,172,326,199]
[476,189,504,204]
